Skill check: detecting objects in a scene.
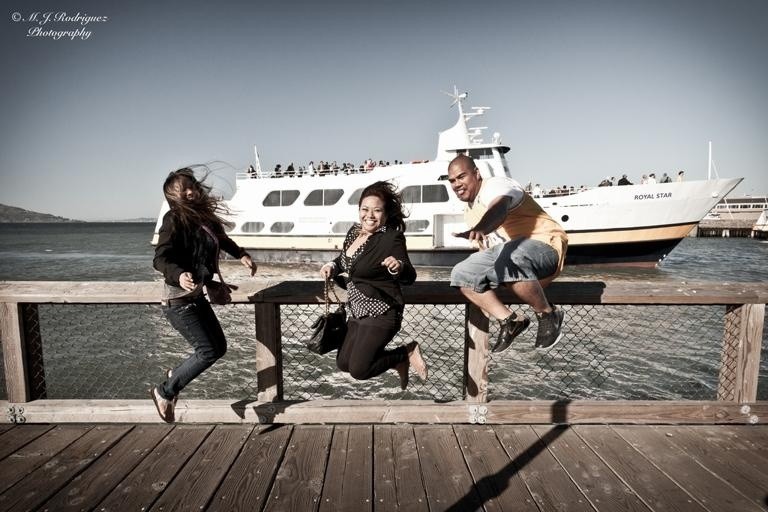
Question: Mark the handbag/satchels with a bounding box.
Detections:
[308,275,348,355]
[205,279,239,305]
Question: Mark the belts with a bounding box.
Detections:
[161,295,199,306]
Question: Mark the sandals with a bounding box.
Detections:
[395,364,409,390]
[150,369,180,425]
[408,341,428,383]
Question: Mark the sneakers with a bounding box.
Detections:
[535,303,565,351]
[490,312,531,355]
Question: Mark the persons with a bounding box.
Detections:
[599,171,684,186]
[526,181,585,198]
[248,158,403,179]
[448,156,568,354]
[151,168,257,423]
[320,180,428,390]
